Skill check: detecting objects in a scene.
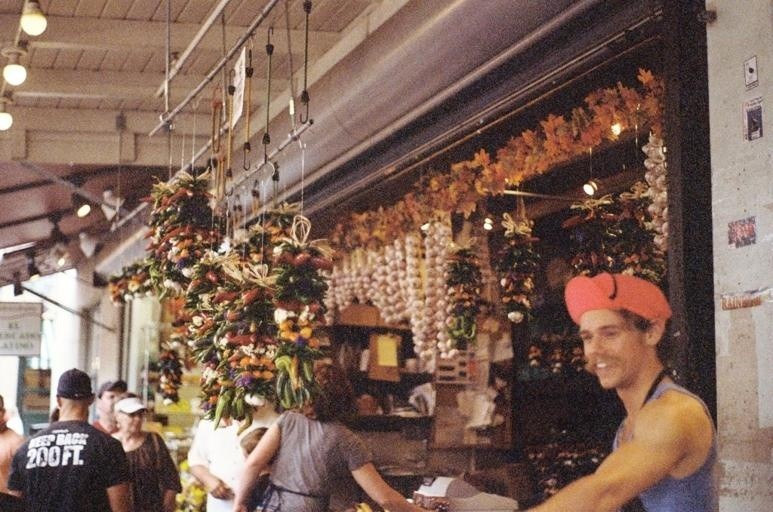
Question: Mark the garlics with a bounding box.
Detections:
[323,222,460,364]
[642,134,669,252]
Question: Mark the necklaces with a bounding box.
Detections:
[640,368,677,411]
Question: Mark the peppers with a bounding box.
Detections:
[136,186,331,436]
[452,315,479,340]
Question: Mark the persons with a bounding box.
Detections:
[234,365,432,512]
[528,273,719,512]
[0,368,181,512]
[188,409,280,512]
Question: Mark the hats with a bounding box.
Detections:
[566,273,672,328]
[98,380,127,398]
[115,396,150,416]
[57,369,93,399]
